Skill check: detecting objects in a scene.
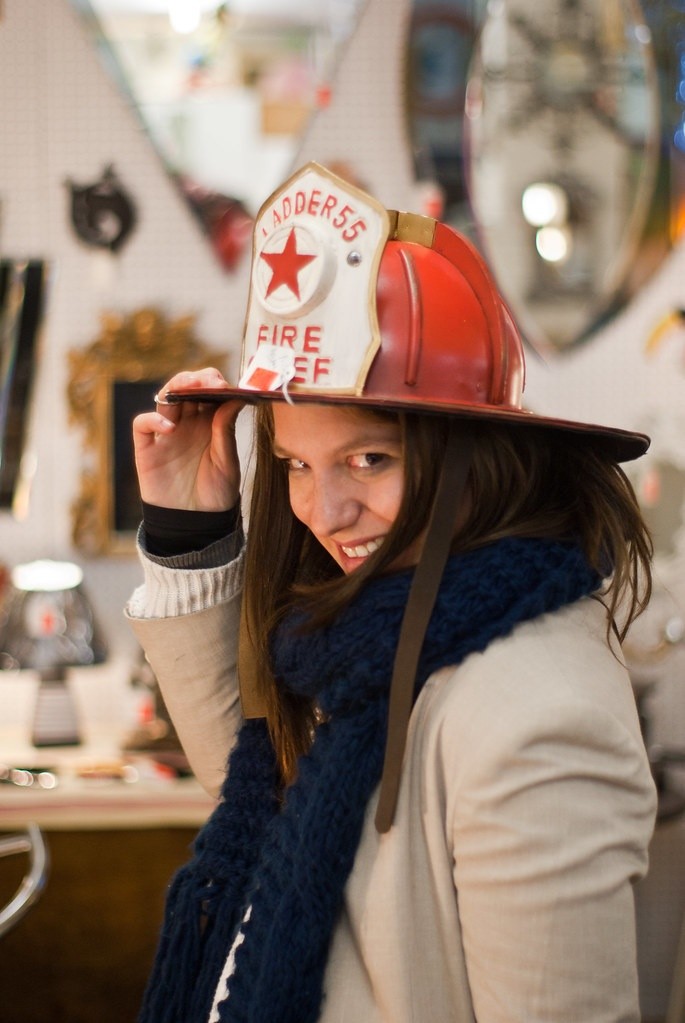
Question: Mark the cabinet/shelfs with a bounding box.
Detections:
[0,654,218,1023]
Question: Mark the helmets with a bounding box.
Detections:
[166,160,651,465]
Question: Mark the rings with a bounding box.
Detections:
[154,394,181,405]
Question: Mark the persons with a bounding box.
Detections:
[131,161,658,1023]
